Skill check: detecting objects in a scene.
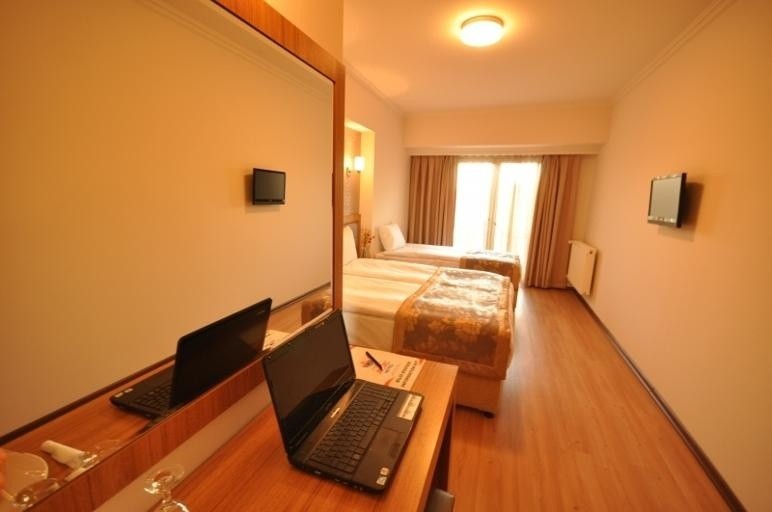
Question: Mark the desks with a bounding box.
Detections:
[95,348,463,512]
[0,325,290,512]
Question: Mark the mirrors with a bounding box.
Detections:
[1,0,345,443]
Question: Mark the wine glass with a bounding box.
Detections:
[144,464,193,512]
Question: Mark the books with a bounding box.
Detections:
[351,346,426,392]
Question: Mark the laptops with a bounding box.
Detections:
[263,308,426,492]
[110,297,272,420]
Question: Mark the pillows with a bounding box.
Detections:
[378,223,406,250]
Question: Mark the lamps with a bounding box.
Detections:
[458,14,509,51]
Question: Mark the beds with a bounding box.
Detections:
[342,212,515,419]
[370,230,521,292]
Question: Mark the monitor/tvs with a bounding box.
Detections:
[648,172,687,228]
[254,167,286,204]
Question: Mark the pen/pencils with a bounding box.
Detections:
[366,350,383,370]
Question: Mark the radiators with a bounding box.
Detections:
[566,239,597,297]
